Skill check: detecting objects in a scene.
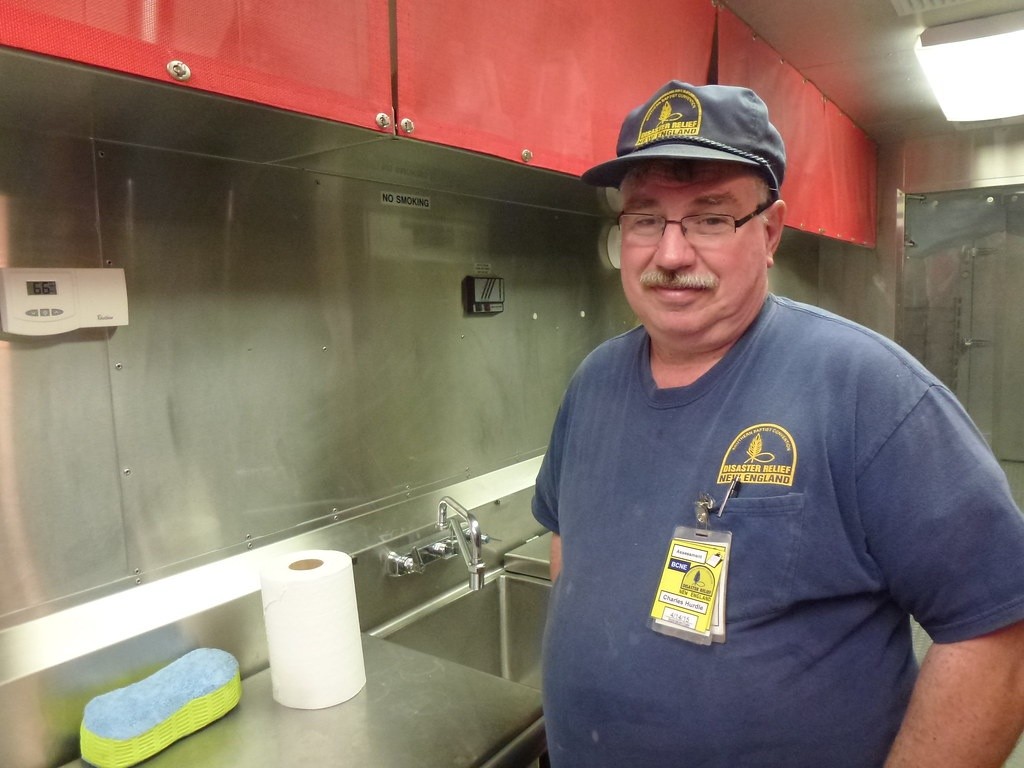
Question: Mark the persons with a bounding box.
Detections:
[532,78,1024,768]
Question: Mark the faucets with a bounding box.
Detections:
[383,496,492,591]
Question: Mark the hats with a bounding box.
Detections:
[580,80,784,199]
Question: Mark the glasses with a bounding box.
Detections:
[616,200,775,242]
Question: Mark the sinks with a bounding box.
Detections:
[372,573,554,696]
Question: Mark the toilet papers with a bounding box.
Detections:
[259,548,369,707]
